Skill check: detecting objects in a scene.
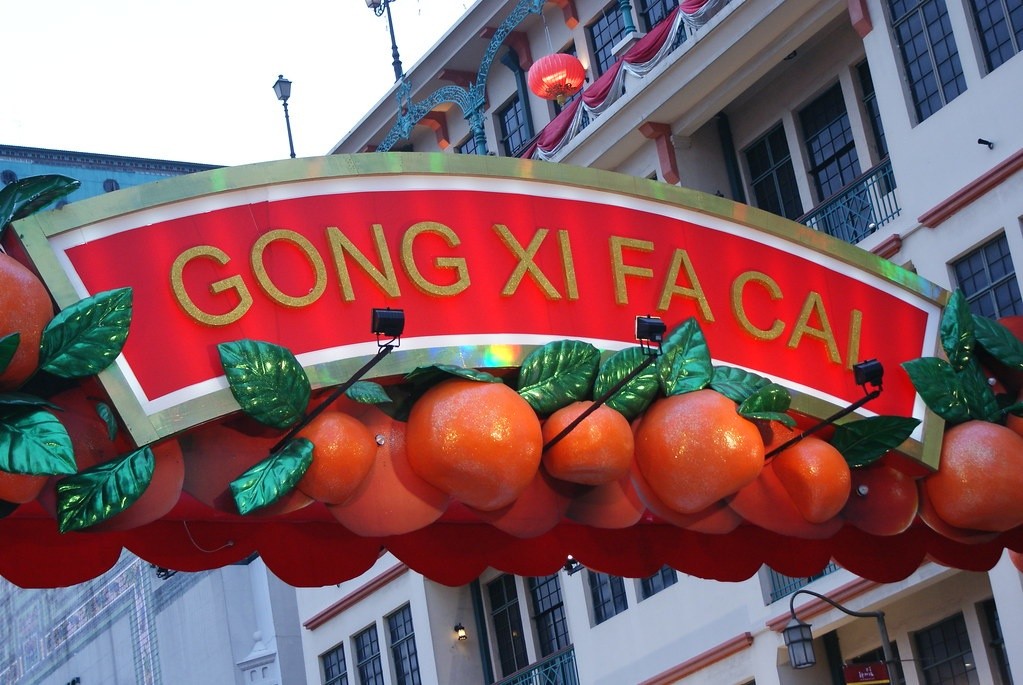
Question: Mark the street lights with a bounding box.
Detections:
[781,590,901,685]
[365,0,403,83]
[272,74,296,159]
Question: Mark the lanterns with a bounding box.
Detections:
[527,53,586,105]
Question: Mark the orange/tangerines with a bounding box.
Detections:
[0,252,1023,542]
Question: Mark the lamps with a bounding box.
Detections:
[259,308,406,454]
[751,359,883,458]
[454,623,467,640]
[539,314,667,453]
